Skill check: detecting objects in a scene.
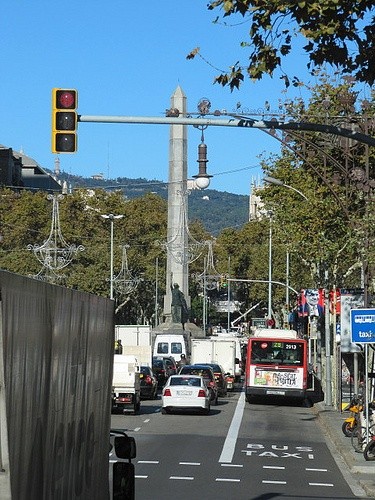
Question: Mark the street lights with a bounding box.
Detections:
[100,214,124,301]
[165,97,375,308]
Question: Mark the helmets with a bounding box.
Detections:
[181,354,185,359]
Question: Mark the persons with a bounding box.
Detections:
[170,272,189,323]
[176,355,190,373]
[297,288,324,316]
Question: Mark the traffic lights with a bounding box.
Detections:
[220,271,227,288]
[50,88,78,153]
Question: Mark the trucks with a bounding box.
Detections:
[0,266,137,500]
[115,320,299,391]
[111,352,143,414]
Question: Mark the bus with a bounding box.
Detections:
[244,335,310,404]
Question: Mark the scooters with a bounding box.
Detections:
[342,392,375,461]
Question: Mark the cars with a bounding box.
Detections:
[193,363,230,398]
[161,375,212,415]
[178,365,220,406]
[137,365,158,400]
